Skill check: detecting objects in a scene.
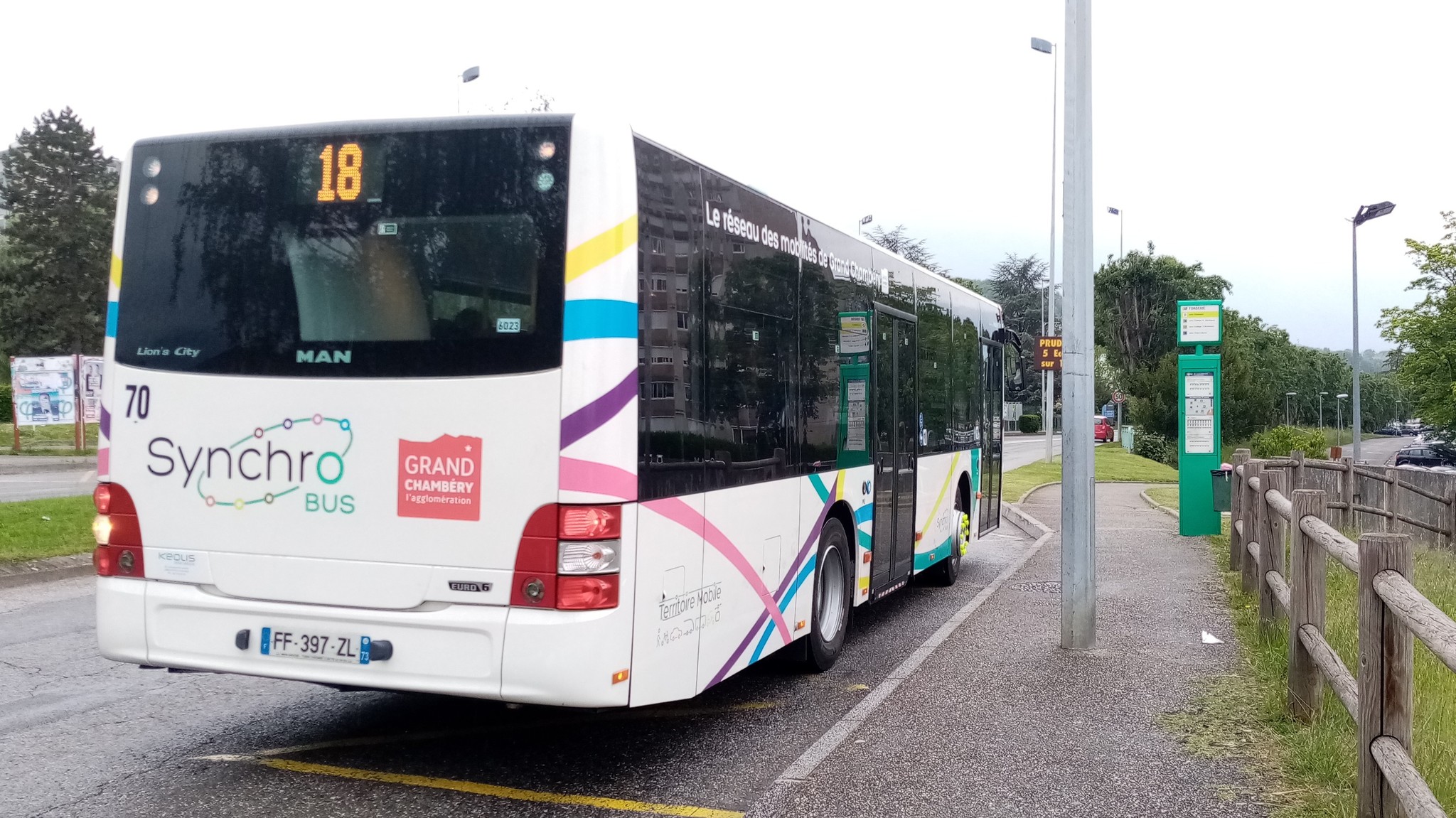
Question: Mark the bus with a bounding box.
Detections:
[91,106,1027,709]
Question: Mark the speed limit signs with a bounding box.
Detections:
[1112,390,1126,403]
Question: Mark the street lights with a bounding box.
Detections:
[1351,201,1396,462]
[1286,392,1297,427]
[1319,391,1329,431]
[1109,207,1123,260]
[1395,400,1402,435]
[1336,393,1349,447]
[1031,36,1057,466]
[859,214,872,236]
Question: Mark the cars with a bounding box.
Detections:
[1374,418,1456,467]
[1094,416,1114,442]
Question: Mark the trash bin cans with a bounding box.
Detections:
[1211,470,1231,511]
